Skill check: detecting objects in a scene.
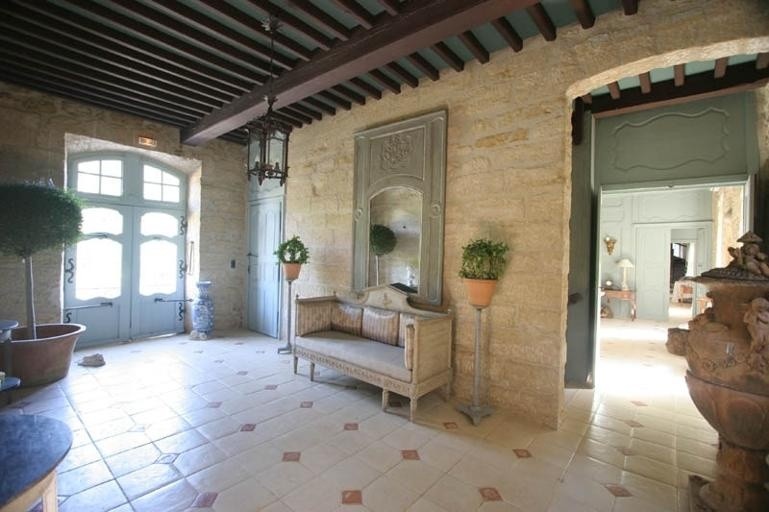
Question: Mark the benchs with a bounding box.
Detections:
[289,283,452,422]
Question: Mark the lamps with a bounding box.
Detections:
[242,13,291,186]
[616,258,634,291]
[604,236,617,256]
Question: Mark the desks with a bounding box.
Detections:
[675,280,693,304]
[0,413,73,511]
[601,288,637,323]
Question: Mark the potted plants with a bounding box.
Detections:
[273,234,310,282]
[459,237,512,307]
[0,180,87,389]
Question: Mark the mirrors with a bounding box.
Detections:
[353,106,447,307]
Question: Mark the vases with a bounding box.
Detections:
[190,282,214,336]
